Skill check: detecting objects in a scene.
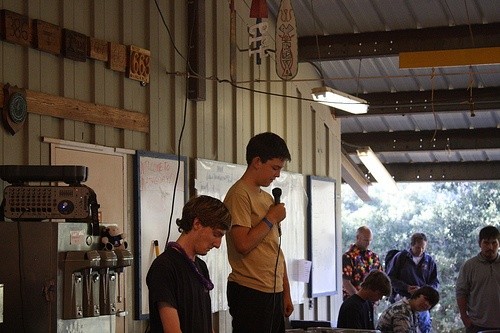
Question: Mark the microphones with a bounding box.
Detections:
[273,188,282,236]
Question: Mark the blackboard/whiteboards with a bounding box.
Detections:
[194,156,308,317]
[135,150,190,319]
[306,172,339,297]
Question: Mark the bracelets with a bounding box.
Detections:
[261,216,275,231]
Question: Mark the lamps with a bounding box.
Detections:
[357,145,396,186]
[311,58,370,115]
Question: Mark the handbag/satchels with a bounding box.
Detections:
[384,249,404,304]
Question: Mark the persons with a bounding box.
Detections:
[145,194,233,332]
[221,131,293,333]
[456,226,500,333]
[336,268,392,330]
[387,232,440,333]
[377,284,440,333]
[341,225,384,300]
